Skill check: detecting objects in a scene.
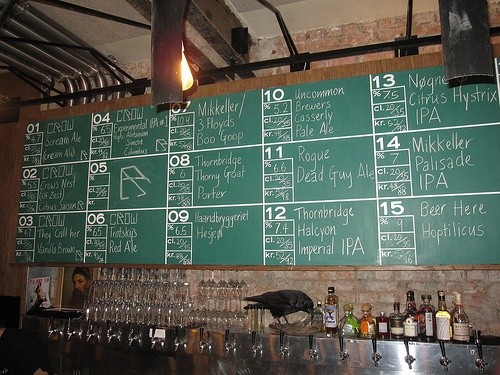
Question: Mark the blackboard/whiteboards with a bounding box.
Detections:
[10,59,500,271]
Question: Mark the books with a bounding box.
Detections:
[29,277,52,308]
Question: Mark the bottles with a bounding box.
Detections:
[389,301,404,339]
[417,294,436,341]
[402,290,420,341]
[339,303,376,340]
[436,290,453,341]
[311,300,324,333]
[451,289,472,343]
[324,286,340,335]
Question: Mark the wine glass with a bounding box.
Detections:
[376,311,391,339]
[84,265,250,329]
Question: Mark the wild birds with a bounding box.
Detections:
[241,289,316,325]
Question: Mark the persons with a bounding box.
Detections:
[35,282,43,299]
[0,317,54,375]
[68,267,93,308]
